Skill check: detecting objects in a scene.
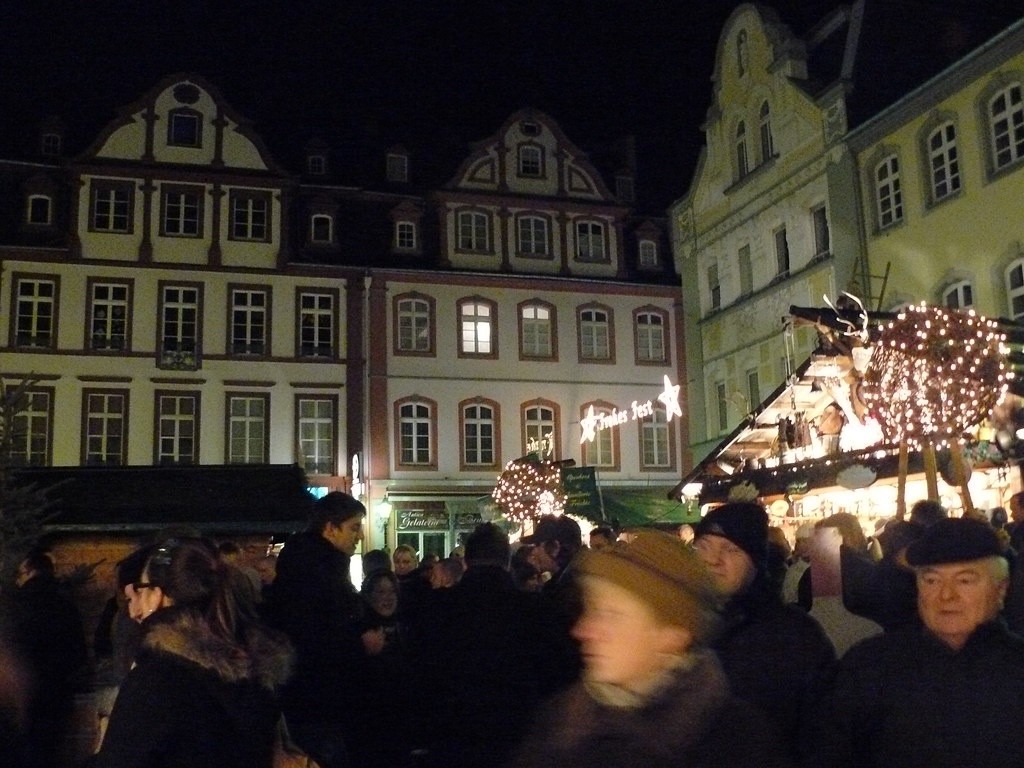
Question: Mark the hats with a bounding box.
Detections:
[698,502,768,574]
[907,518,1005,565]
[573,527,723,651]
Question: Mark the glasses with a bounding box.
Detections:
[132,582,151,595]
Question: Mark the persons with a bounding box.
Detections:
[1,490,1024,768]
[831,518,1023,767]
